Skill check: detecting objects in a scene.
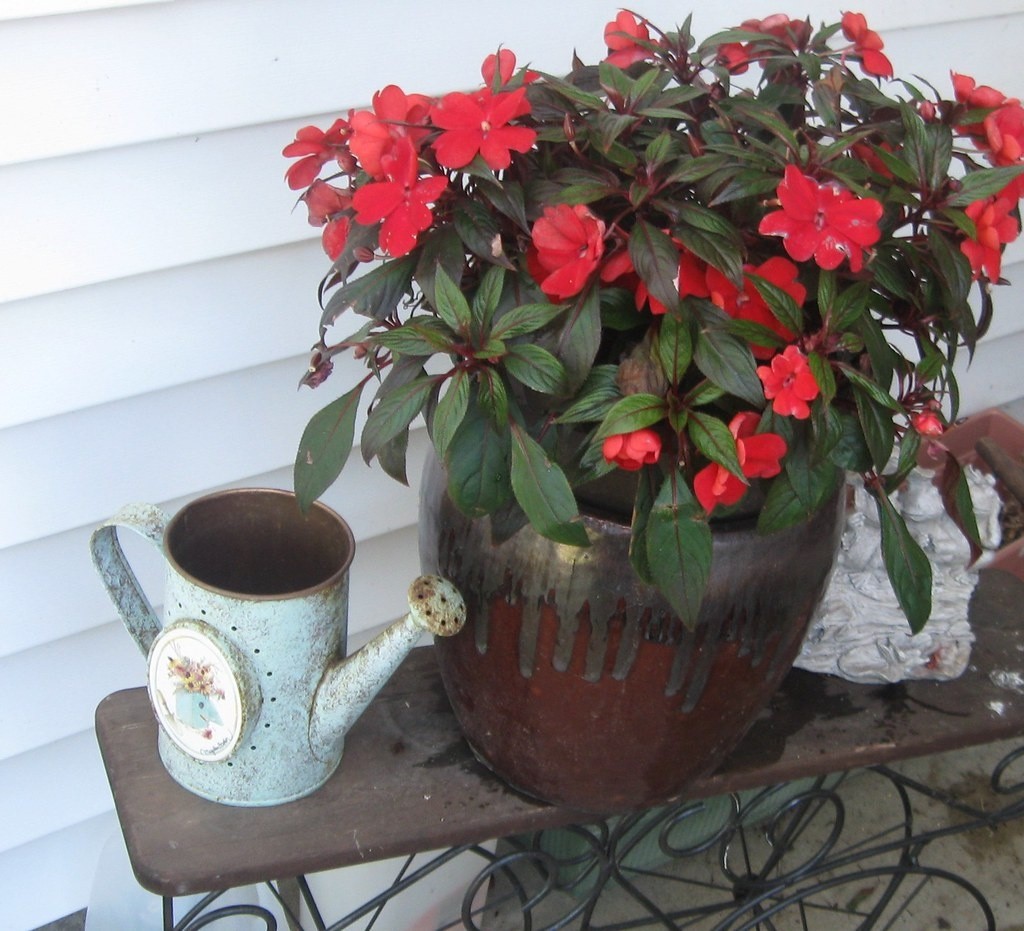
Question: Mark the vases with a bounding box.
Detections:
[418,422,845,811]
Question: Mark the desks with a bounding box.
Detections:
[94,645,1023,931]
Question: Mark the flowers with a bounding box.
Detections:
[285,6,1024,627]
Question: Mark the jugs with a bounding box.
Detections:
[89,487,467,809]
